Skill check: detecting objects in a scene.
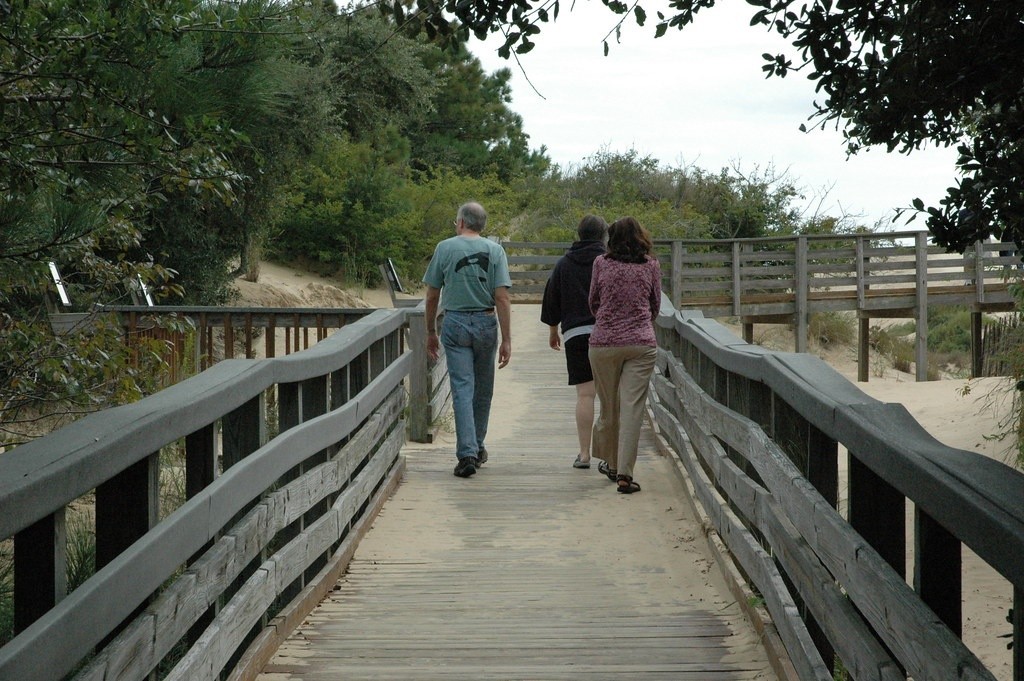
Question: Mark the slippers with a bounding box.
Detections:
[572,455,590,467]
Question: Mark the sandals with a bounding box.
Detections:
[598,460,617,479]
[617,475,641,493]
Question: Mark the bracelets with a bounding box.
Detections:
[426,328,436,336]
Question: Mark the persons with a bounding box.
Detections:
[589,218,661,493]
[422,202,512,476]
[540,214,608,468]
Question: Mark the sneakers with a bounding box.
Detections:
[454,457,477,478]
[475,448,488,467]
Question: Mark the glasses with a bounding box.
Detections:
[453,220,458,226]
